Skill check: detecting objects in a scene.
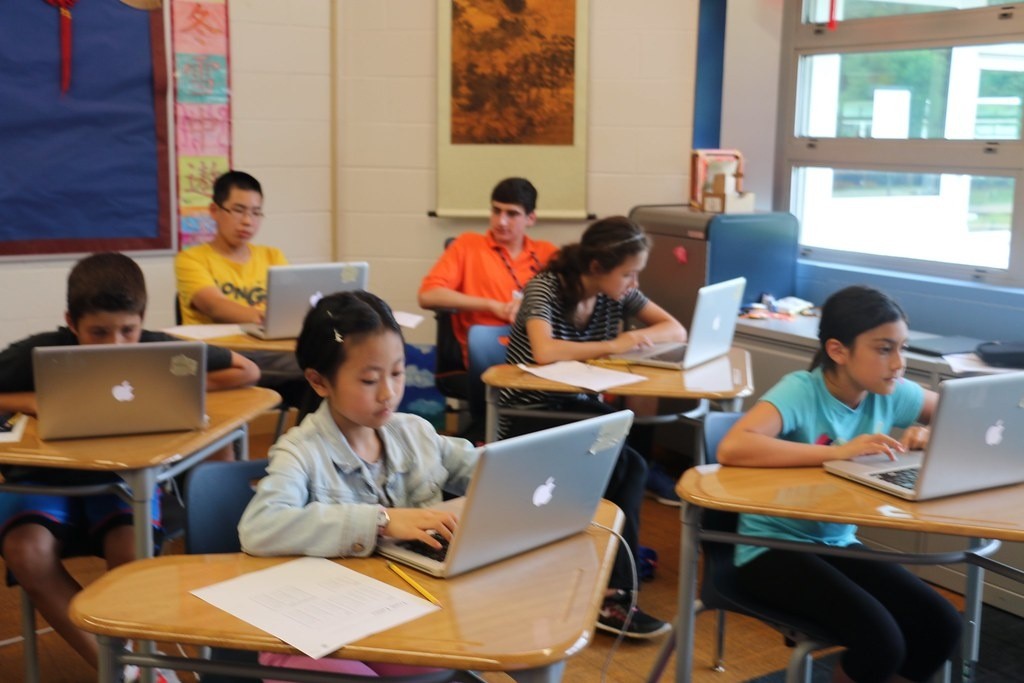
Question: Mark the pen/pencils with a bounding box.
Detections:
[588,359,637,366]
[384,559,444,609]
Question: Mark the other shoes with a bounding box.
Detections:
[116,638,181,683]
[644,470,683,506]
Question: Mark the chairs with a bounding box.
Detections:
[467,327,517,443]
[184,455,274,682]
[644,414,849,683]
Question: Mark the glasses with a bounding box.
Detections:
[215,204,263,219]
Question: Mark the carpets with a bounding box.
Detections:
[744,601,1022,683]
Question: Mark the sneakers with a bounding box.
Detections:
[595,593,672,639]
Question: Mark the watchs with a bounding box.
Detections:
[376,507,390,536]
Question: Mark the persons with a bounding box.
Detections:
[177,168,330,414]
[0,250,260,682]
[418,175,564,434]
[238,292,478,683]
[721,285,970,683]
[494,217,688,641]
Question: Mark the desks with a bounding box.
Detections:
[675,465,1023,683]
[478,344,755,470]
[155,320,316,426]
[70,498,626,683]
[1,387,288,683]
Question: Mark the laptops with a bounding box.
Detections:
[823,371,1024,503]
[374,409,635,580]
[33,342,207,443]
[240,262,369,340]
[609,277,747,370]
